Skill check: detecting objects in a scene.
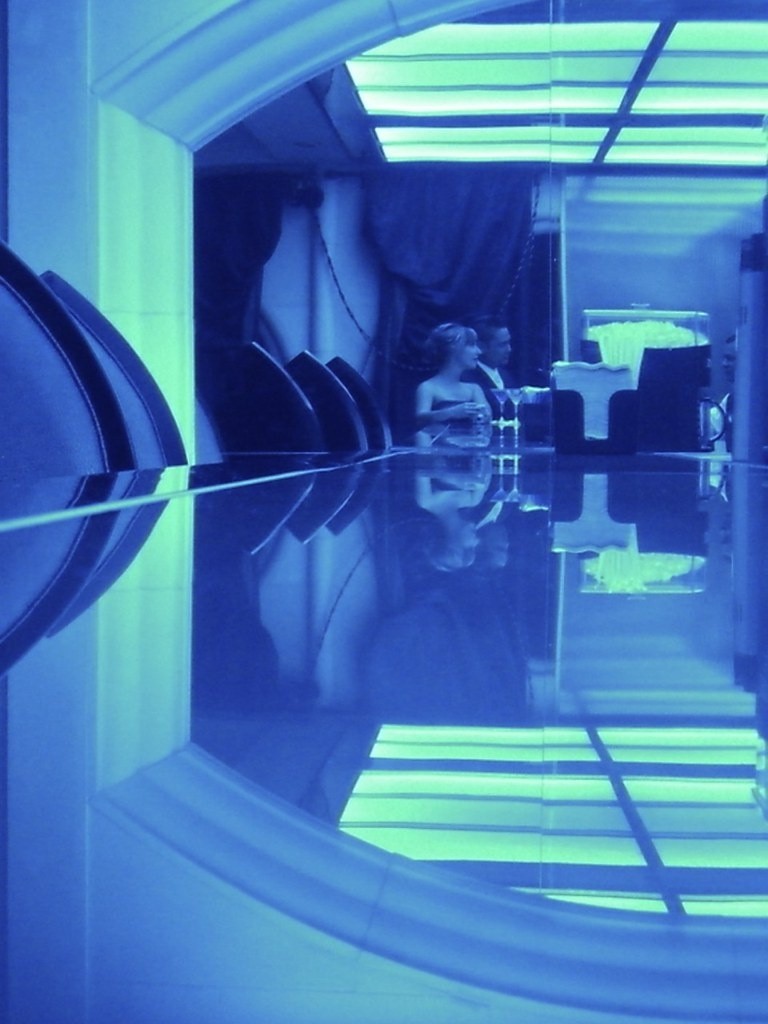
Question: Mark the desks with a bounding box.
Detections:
[0,450,768,1024]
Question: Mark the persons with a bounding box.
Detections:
[414,315,517,422]
[414,450,510,572]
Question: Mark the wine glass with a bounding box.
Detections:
[505,388,522,426]
[491,389,508,426]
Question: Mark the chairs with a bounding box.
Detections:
[605,386,701,450]
[248,343,395,456]
[0,241,190,482]
[513,388,588,452]
